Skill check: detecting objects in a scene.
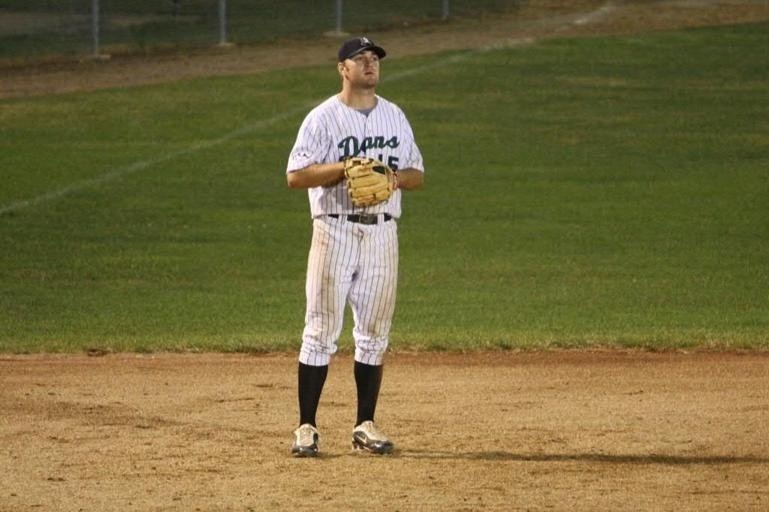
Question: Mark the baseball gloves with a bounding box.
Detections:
[343,156,398,206]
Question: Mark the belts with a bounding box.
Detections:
[328,213,392,224]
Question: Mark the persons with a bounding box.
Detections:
[286,36,425,457]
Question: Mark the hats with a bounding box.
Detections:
[338,37,386,61]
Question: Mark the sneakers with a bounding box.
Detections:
[352,419,393,455]
[292,423,320,457]
